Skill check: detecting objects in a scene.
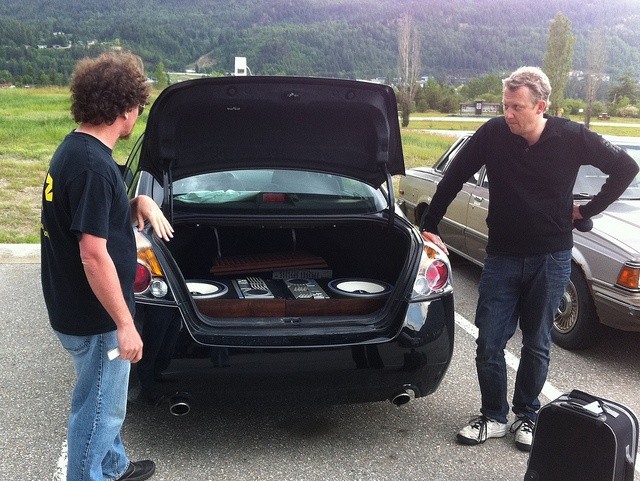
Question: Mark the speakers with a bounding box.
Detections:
[283,276,392,316]
[186,276,287,318]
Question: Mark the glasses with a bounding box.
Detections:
[135,101,145,116]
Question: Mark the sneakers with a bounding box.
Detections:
[510,413,535,451]
[457,414,507,443]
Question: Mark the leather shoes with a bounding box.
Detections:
[118,460,155,481]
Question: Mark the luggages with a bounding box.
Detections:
[523,390,639,481]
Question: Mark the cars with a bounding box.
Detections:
[111,77,454,417]
[400,133,640,349]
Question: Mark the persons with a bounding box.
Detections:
[39,52,174,481]
[418,65,640,454]
